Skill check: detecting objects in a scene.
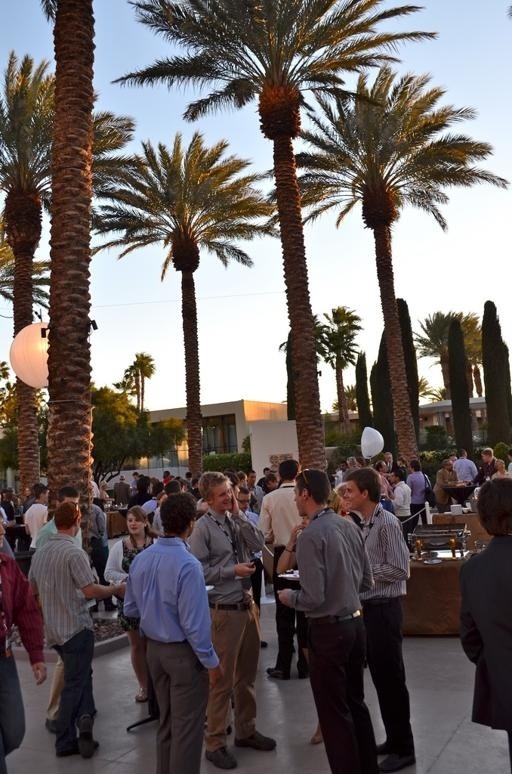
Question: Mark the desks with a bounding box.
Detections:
[432,512,494,548]
[104,512,130,537]
[399,550,471,637]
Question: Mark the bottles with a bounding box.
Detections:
[449,535,456,560]
[415,539,422,561]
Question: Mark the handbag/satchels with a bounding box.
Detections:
[424,487,435,508]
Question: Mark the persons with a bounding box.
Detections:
[457,477,511,752]
[432,449,510,513]
[257,452,430,679]
[277,492,344,745]
[1,468,276,772]
[276,467,378,771]
[345,467,417,771]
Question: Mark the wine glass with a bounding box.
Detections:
[103,503,111,513]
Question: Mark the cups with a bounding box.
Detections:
[450,504,462,515]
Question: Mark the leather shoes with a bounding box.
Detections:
[136,687,276,769]
[43,713,99,759]
[377,741,415,771]
[267,666,308,680]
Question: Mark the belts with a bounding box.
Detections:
[309,610,360,624]
[209,602,252,610]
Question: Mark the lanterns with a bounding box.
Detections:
[10,322,50,389]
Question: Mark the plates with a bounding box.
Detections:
[205,585,215,592]
[276,573,300,582]
[424,559,442,564]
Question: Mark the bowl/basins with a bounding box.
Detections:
[462,507,470,514]
